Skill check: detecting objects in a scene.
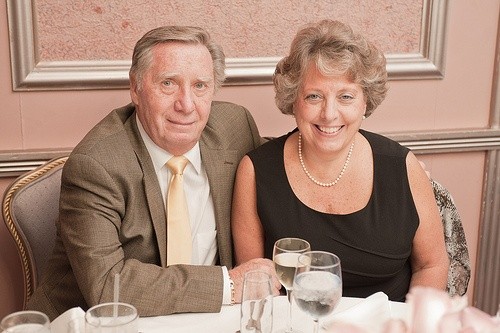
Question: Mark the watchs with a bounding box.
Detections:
[230,277,236,305]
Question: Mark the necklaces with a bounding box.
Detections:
[298,131,355,186]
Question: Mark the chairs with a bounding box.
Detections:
[1,156,71,311]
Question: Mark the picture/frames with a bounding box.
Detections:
[6,0,448,92]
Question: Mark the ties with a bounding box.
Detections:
[165,155,194,267]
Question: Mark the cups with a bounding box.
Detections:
[85,302,137,332]
[0,310,51,333]
[240,270,274,333]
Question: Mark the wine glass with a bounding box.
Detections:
[273,238,311,333]
[292,251,342,333]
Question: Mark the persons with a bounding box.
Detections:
[231,20,448,302]
[26,25,282,322]
